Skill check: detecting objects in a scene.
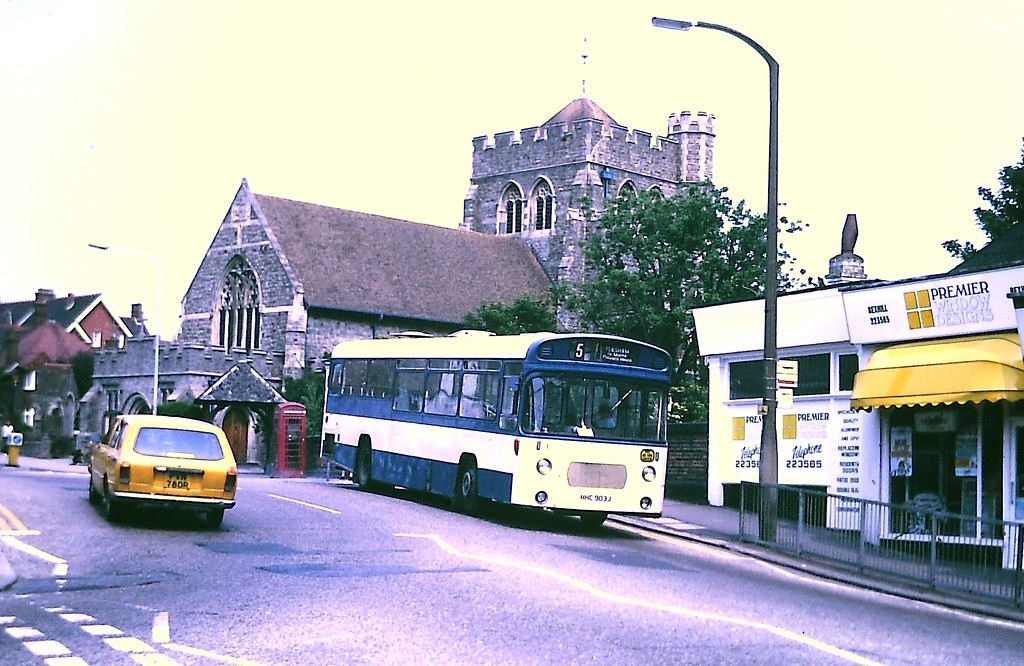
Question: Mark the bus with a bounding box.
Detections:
[317,324,673,526]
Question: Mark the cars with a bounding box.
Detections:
[83,415,237,526]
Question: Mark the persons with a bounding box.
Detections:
[1,417,15,455]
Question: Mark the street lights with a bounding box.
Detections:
[651,13,781,544]
